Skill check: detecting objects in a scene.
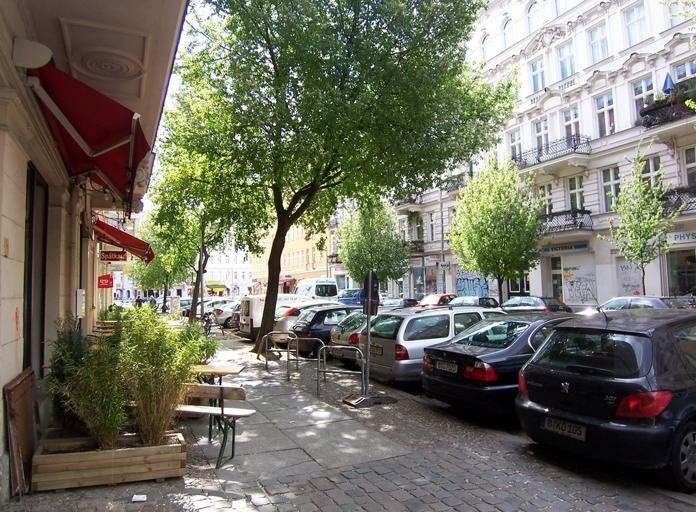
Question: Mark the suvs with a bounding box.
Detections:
[231,301,241,328]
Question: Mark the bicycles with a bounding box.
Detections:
[195,309,213,340]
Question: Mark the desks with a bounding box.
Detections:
[189,364,246,445]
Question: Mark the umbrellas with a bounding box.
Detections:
[661,71,678,94]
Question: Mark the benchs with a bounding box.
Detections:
[123,383,256,470]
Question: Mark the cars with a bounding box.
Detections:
[383,297,418,306]
[359,306,505,382]
[424,312,574,406]
[338,288,365,304]
[515,310,695,487]
[595,295,668,311]
[273,299,344,344]
[501,296,573,313]
[290,305,362,352]
[380,290,387,304]
[175,292,232,328]
[329,309,390,360]
[415,293,456,305]
[447,297,498,305]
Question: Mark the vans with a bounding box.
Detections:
[294,277,336,300]
[235,295,316,341]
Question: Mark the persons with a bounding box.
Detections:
[136,295,157,307]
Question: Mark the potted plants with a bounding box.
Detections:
[30,312,223,496]
